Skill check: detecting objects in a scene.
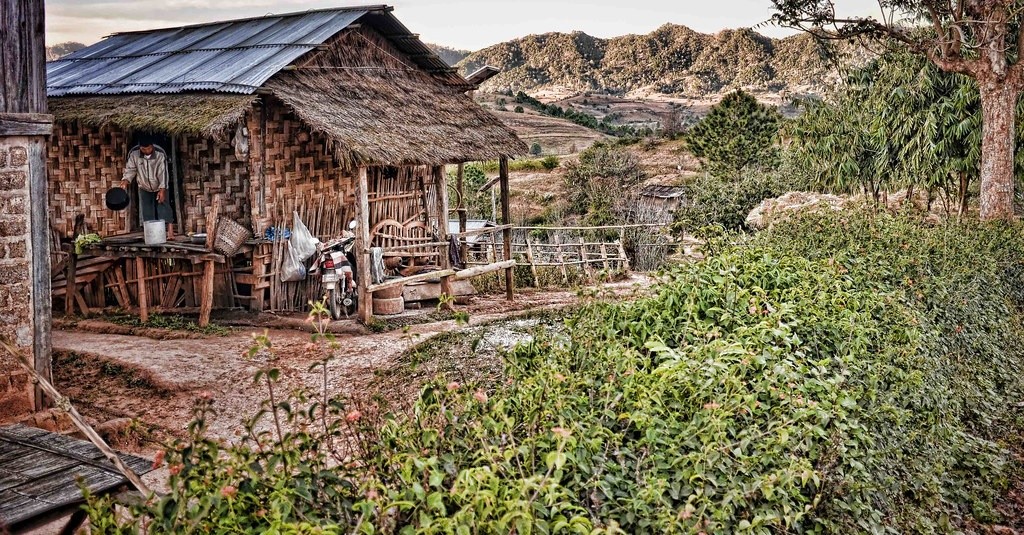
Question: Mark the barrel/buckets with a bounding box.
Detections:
[143,218,167,244]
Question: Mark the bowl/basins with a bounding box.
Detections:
[190,233,207,244]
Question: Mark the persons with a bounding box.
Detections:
[119,137,176,241]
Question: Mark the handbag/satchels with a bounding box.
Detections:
[280,210,320,283]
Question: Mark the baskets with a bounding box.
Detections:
[214,216,252,258]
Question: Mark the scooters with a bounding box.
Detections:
[310,219,358,321]
